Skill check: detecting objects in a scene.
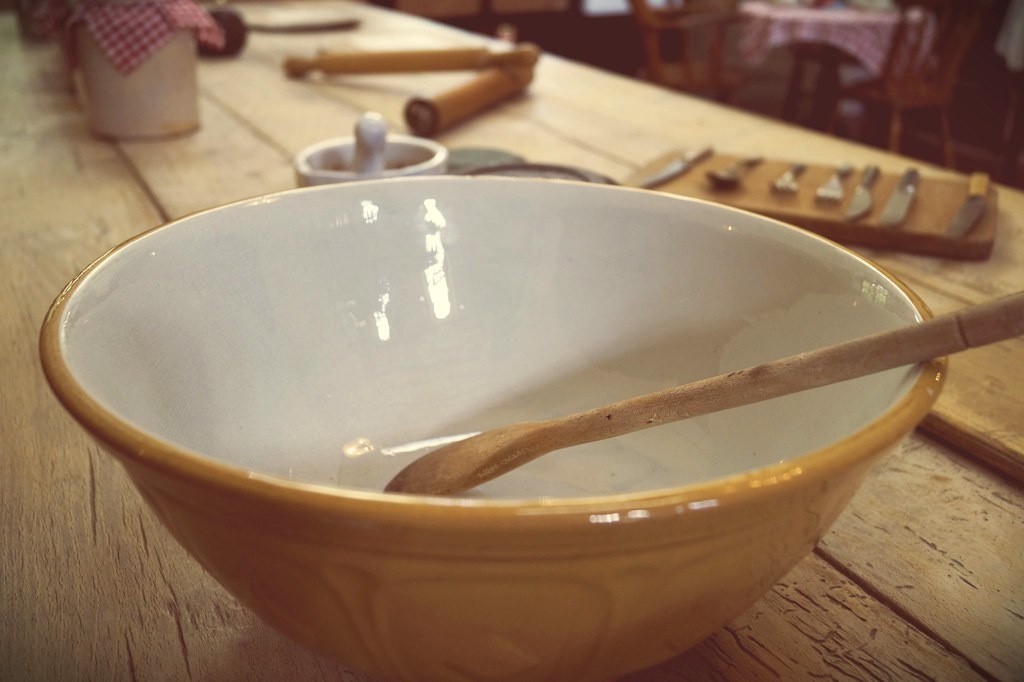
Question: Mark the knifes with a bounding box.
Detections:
[950,170,989,239]
[846,165,880,218]
[637,147,710,190]
[879,170,920,226]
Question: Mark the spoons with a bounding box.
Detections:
[705,158,762,188]
[817,165,855,201]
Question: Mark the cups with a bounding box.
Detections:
[78,25,201,137]
[295,134,451,187]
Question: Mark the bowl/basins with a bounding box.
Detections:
[39,176,948,682]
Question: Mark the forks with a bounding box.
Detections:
[768,166,804,194]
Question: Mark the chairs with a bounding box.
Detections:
[826,0,990,169]
[625,0,753,116]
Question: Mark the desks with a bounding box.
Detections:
[0,0,1024,682]
